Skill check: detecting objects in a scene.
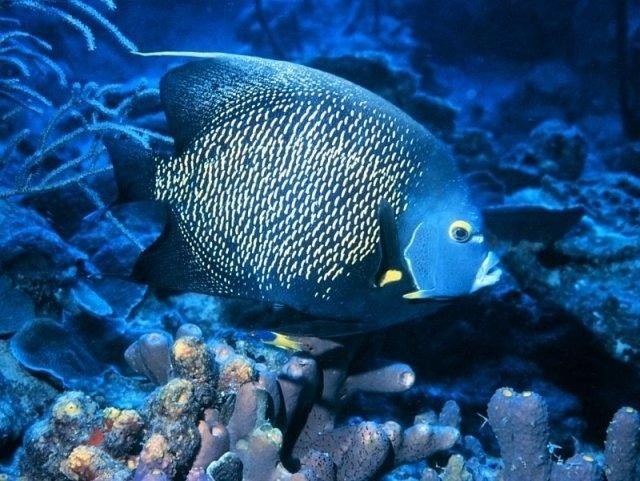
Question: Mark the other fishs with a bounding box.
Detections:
[101,53,503,340]
